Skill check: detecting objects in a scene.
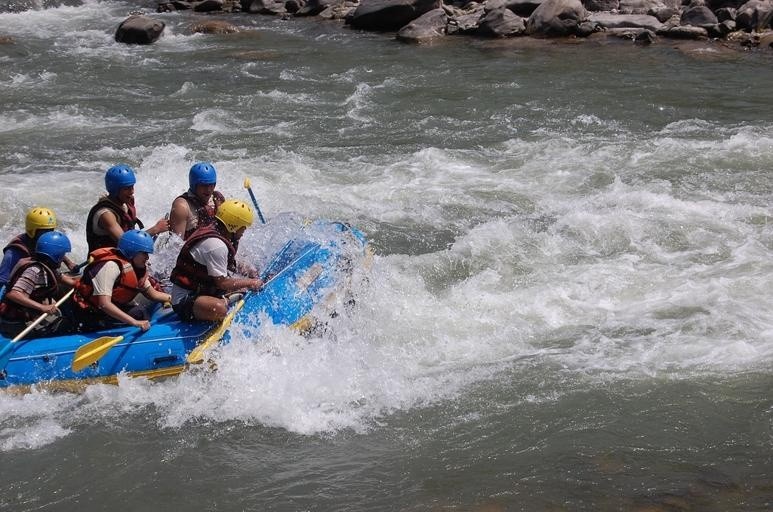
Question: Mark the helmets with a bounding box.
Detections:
[188,161,217,192]
[25,207,56,237]
[104,164,136,193]
[117,228,155,259]
[35,228,72,265]
[215,198,255,235]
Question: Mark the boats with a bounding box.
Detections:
[0,216,373,396]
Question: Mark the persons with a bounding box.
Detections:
[170,163,224,241]
[171,200,265,322]
[83,165,170,260]
[71,230,172,331]
[1,232,75,339]
[0,208,80,295]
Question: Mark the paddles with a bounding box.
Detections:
[73,311,175,371]
[0,287,75,371]
[187,218,312,364]
